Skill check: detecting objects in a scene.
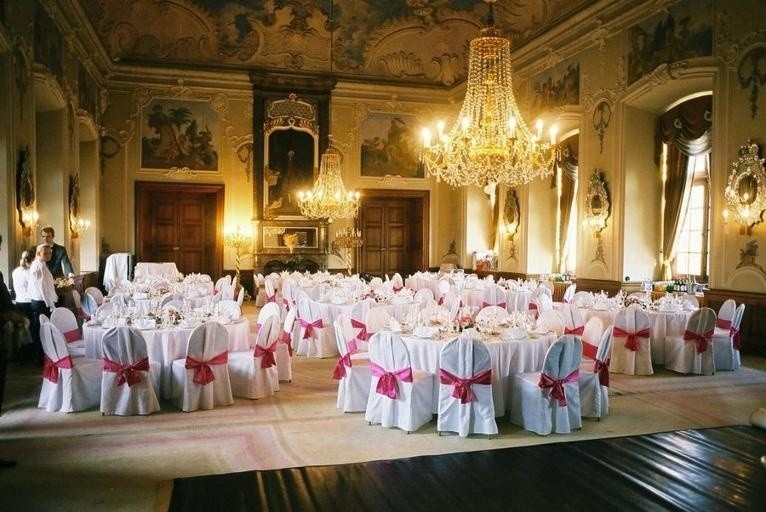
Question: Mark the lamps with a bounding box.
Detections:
[332,226,364,277]
[222,225,252,300]
[293,1,365,220]
[415,0,565,187]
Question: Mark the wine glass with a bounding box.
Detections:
[477,316,496,338]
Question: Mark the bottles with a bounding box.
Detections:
[640,274,703,294]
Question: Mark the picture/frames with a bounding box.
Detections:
[263,93,320,218]
[257,218,326,254]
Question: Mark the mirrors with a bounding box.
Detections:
[68,174,83,237]
[18,145,36,233]
[583,168,611,237]
[725,135,766,232]
[502,188,520,238]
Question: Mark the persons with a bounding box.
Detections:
[278,149,306,197]
[0,237,28,467]
[40,227,75,305]
[27,244,58,366]
[10,250,35,321]
[387,120,402,146]
